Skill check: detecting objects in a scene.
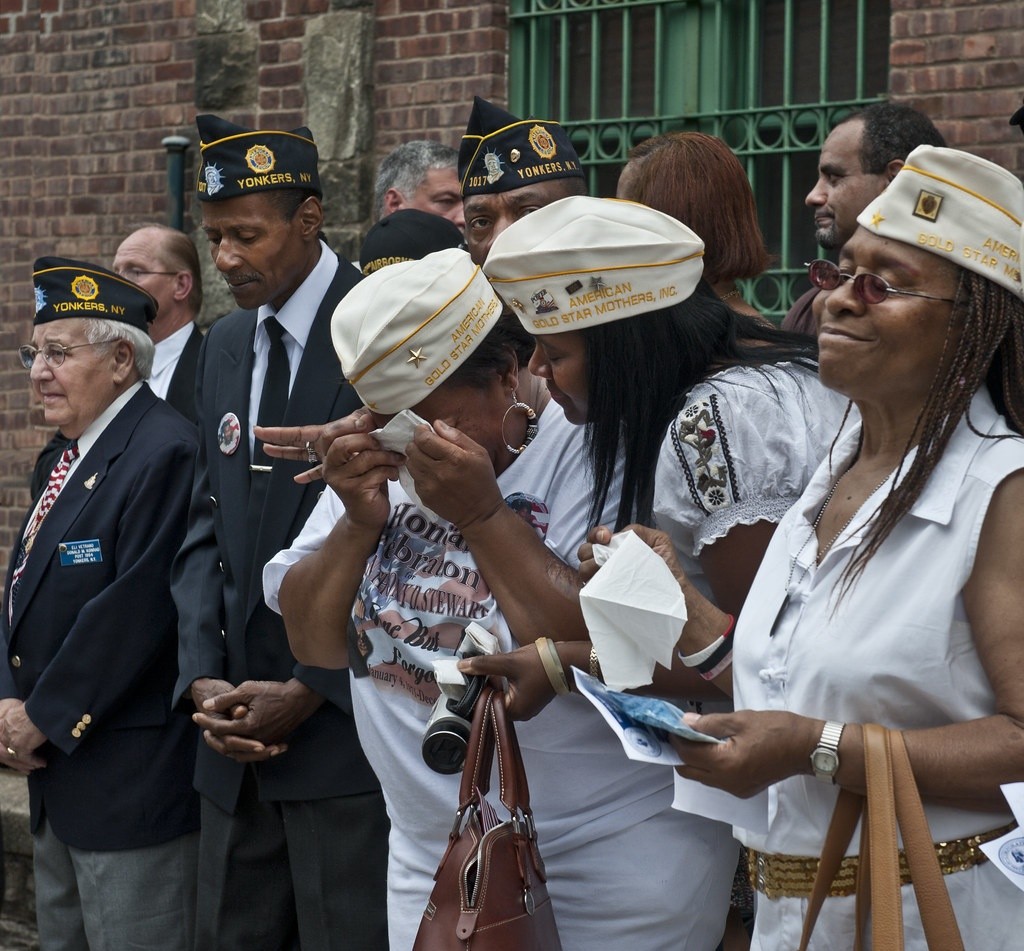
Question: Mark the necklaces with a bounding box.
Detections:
[769,471,894,637]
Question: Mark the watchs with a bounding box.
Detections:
[810,721,846,785]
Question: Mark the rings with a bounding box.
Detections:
[7,748,17,758]
[306,441,317,462]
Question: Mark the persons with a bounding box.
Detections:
[264,248,741,951]
[30,224,206,501]
[617,132,773,330]
[0,257,199,951]
[780,100,947,336]
[169,115,389,951]
[577,144,1024,951]
[219,422,240,454]
[253,196,863,723]
[371,140,468,244]
[360,208,468,275]
[457,96,588,265]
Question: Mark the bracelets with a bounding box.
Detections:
[678,614,738,681]
[535,636,570,695]
[589,648,598,677]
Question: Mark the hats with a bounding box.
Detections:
[32,256,159,334]
[857,144,1024,302]
[330,247,503,414]
[196,114,323,202]
[458,96,585,201]
[359,208,468,277]
[482,195,705,335]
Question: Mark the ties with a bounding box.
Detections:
[8,439,79,627]
[243,316,291,604]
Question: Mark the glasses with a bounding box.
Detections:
[18,338,119,369]
[109,267,178,282]
[804,259,970,306]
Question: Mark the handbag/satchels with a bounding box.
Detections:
[413,686,563,951]
